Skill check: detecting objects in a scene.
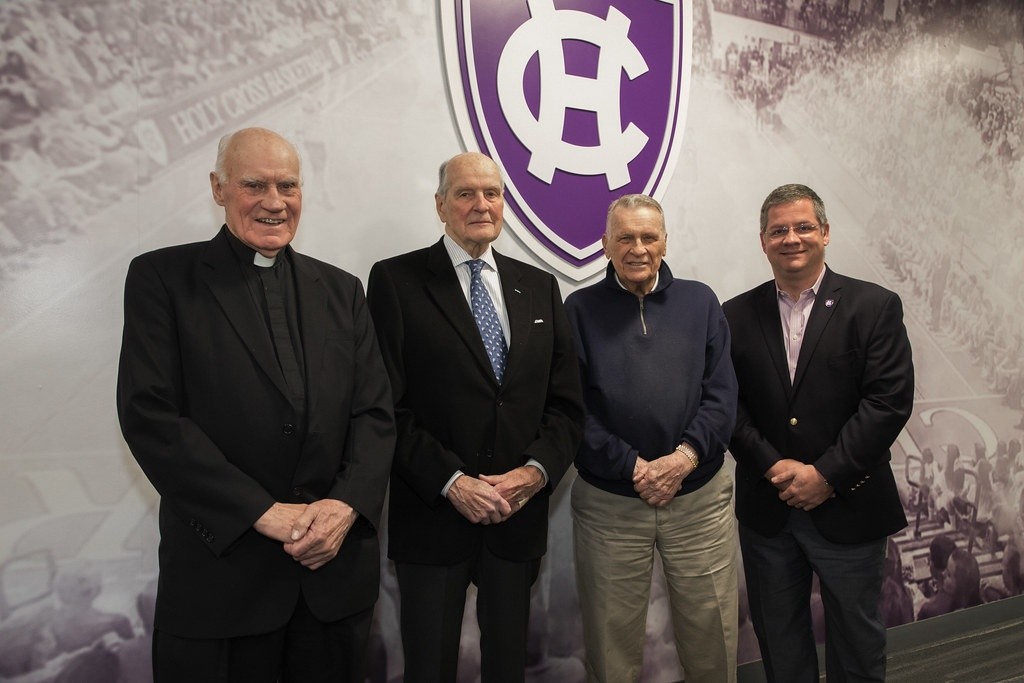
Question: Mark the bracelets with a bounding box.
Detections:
[675,441,700,469]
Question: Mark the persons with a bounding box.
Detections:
[114,124,397,683]
[0,0,440,285]
[2,553,159,683]
[690,0,1024,420]
[884,434,1024,631]
[379,522,753,682]
[720,178,914,683]
[364,149,584,683]
[563,192,748,683]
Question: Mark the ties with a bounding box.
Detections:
[464,259,509,385]
[259,265,307,421]
